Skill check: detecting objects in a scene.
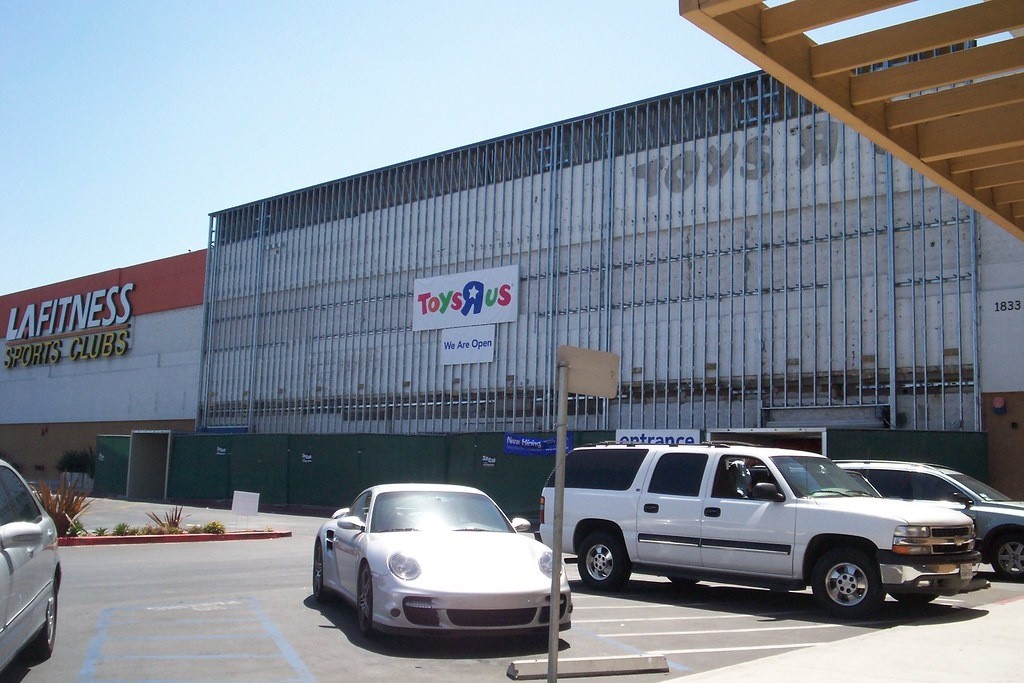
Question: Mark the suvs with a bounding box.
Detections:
[537,440,984,623]
[783,458,1024,584]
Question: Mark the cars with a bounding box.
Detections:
[1,459,61,674]
[312,483,573,640]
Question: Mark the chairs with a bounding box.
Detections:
[729,460,753,498]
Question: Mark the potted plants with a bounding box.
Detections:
[55,445,95,492]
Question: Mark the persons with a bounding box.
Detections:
[728,457,758,499]
